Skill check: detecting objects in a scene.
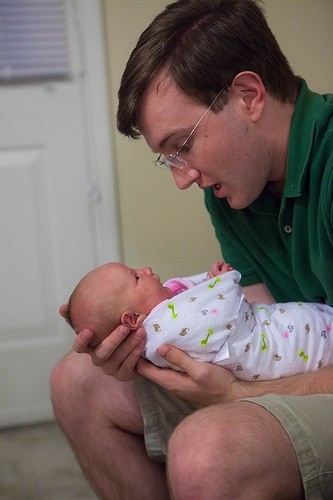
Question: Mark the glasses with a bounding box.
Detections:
[152,80,232,170]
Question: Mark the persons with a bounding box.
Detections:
[50,0,333,499]
[68,261,333,382]
